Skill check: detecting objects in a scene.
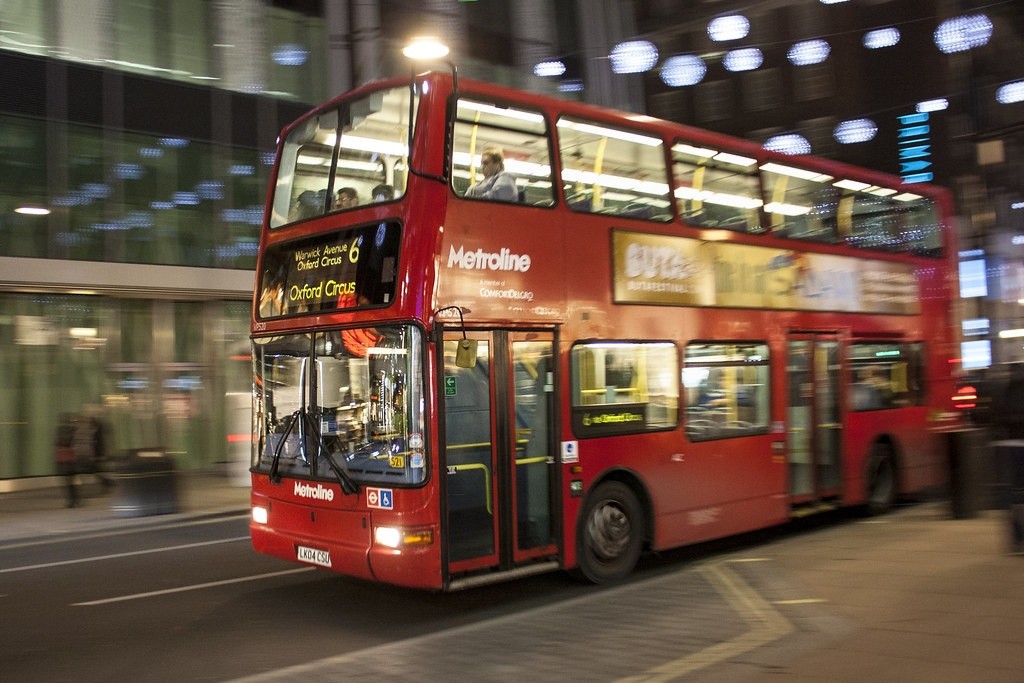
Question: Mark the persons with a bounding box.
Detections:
[371,184,394,202]
[50,405,116,509]
[296,188,358,220]
[605,354,633,395]
[465,149,518,202]
[940,362,1024,557]
[691,369,749,413]
[865,366,893,407]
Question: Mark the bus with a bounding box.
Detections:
[248,59,961,595]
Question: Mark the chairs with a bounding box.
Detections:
[616,382,894,442]
[506,188,946,260]
